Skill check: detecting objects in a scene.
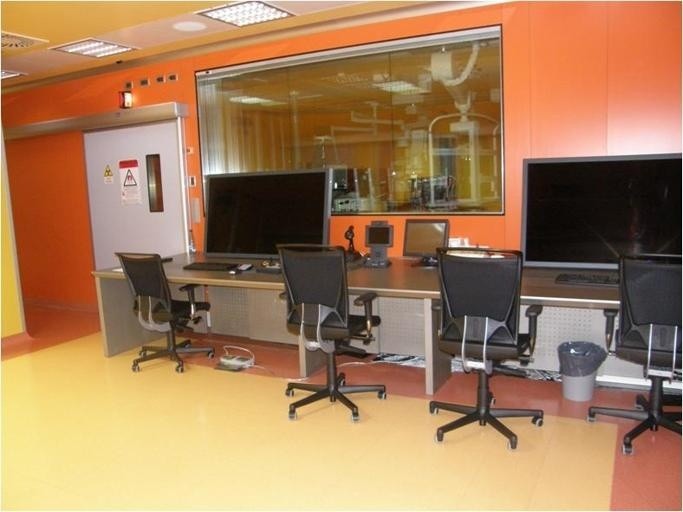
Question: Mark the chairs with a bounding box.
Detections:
[427,246,543,449]
[274,242,388,423]
[586,251,682,456]
[114,250,214,373]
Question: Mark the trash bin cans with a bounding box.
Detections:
[558,341,607,402]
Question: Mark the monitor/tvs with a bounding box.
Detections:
[403,218,450,256]
[203,168,333,260]
[520,153,682,270]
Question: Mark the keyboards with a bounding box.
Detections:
[555,274,619,288]
[183,262,238,271]
[366,224,393,247]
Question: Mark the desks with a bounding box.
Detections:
[90,252,681,360]
